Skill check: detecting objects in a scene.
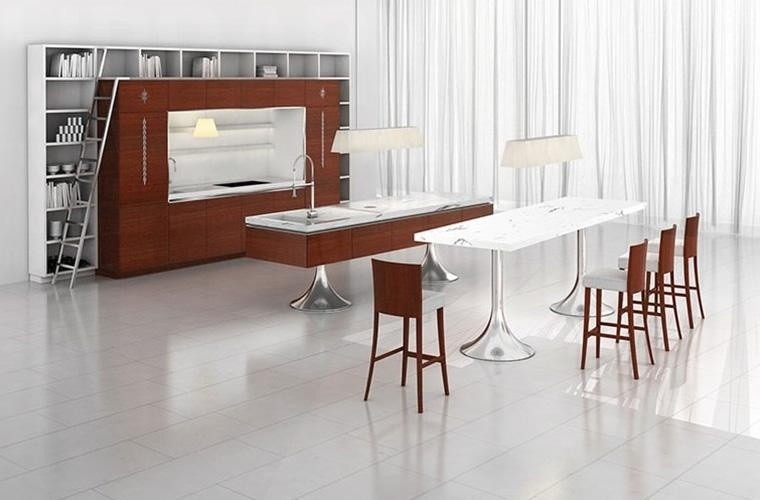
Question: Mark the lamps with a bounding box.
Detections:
[501,135,584,168]
[330,128,424,156]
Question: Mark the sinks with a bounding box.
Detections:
[267,211,351,226]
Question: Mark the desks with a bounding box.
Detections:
[413,199,647,362]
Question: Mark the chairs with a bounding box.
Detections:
[579,215,701,379]
[363,251,448,414]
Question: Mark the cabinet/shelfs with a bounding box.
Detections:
[99,80,353,279]
[98,46,354,80]
[28,46,98,283]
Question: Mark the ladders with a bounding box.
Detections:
[51,77,119,291]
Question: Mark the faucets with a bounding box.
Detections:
[290,154,317,218]
[168,156,176,171]
